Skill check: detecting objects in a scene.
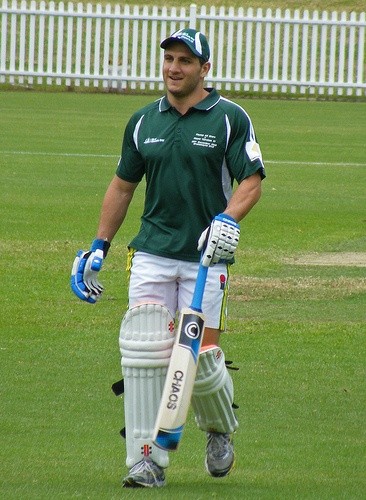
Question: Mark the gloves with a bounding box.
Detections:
[197,213,241,267]
[70,239,110,304]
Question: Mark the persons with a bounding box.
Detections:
[70,29,266,489]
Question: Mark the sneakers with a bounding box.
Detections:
[123,457,166,489]
[205,430,235,477]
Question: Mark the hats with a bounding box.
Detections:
[160,28,210,62]
